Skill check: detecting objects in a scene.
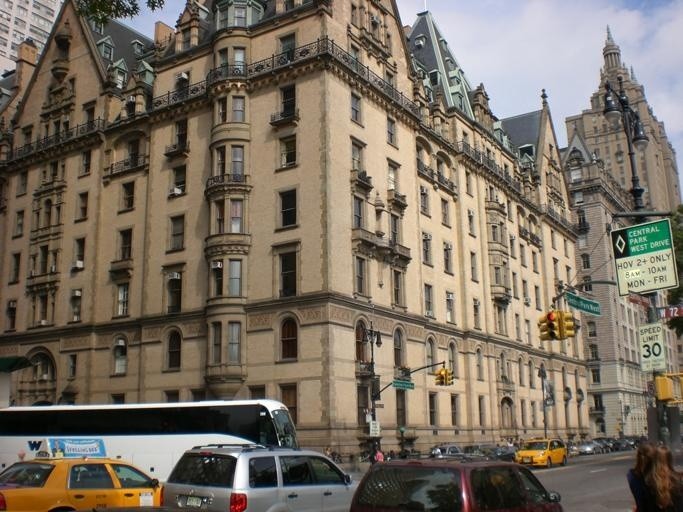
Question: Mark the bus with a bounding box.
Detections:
[1,399,301,484]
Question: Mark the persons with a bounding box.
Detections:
[432,445,442,458]
[499,437,524,446]
[365,447,376,464]
[324,445,333,457]
[51,439,64,458]
[373,446,384,462]
[399,445,410,459]
[644,443,682,512]
[626,440,656,511]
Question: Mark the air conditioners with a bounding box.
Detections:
[118,70,191,103]
[419,186,481,326]
[26,258,227,381]
[168,188,182,198]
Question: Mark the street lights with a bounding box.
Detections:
[362,320,382,450]
[602,75,672,446]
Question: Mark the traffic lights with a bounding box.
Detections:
[434,368,454,386]
[654,374,683,405]
[538,310,575,341]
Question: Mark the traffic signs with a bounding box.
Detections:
[638,323,666,372]
[610,218,680,297]
